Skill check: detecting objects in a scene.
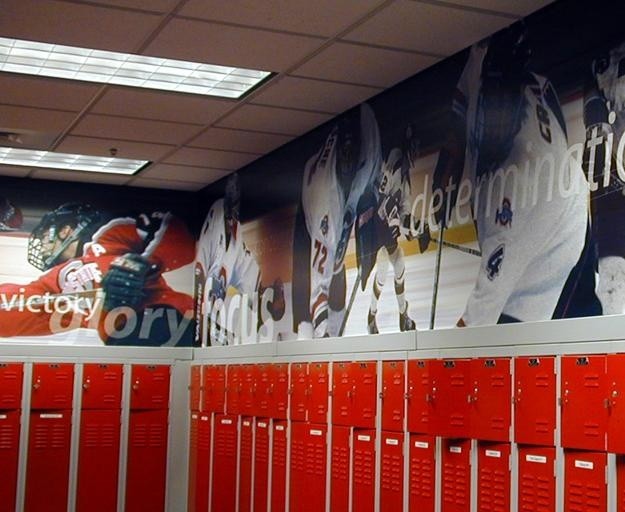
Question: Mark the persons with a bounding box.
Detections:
[1,3,625,348]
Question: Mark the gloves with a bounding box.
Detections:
[101,252,152,308]
[432,147,465,228]
[414,219,431,253]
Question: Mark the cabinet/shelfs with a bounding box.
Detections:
[124,363,171,510]
[71,360,124,510]
[20,363,74,509]
[0,363,23,510]
[188,347,621,512]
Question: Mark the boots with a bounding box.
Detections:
[399,302,416,331]
[367,308,379,334]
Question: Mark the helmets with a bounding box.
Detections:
[336,122,362,188]
[223,170,264,222]
[473,38,526,160]
[28,193,110,271]
